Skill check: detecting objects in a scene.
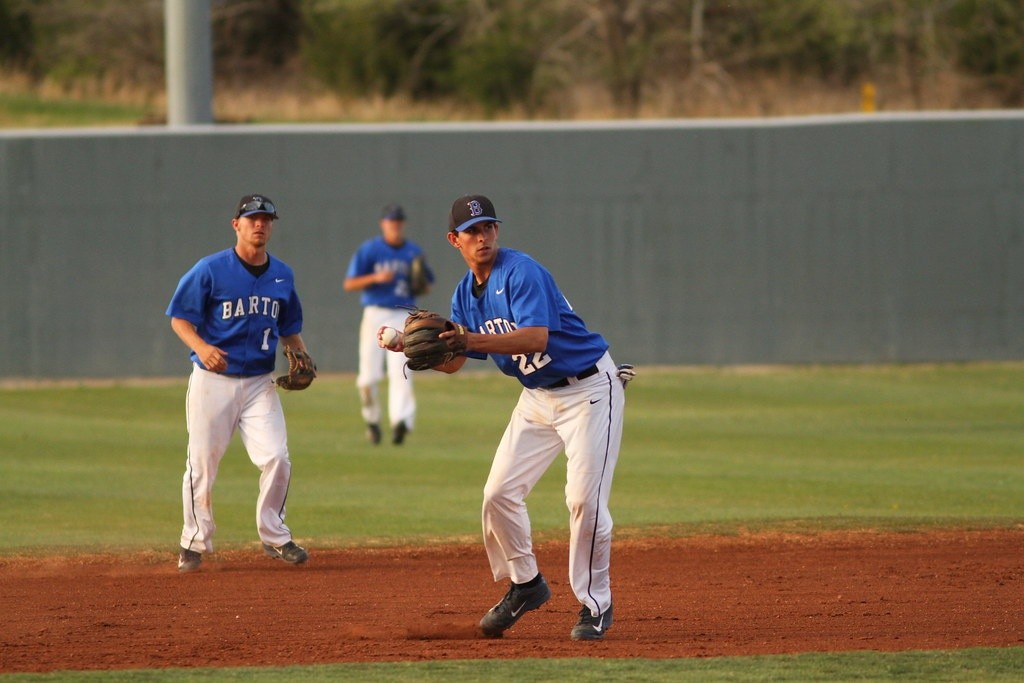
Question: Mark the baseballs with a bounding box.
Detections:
[382,327,399,347]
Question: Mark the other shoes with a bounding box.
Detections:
[367,423,381,443]
[391,420,409,444]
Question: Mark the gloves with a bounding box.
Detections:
[616,363,636,385]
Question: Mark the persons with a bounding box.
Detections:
[376,194,625,640]
[343,202,434,446]
[171,193,318,573]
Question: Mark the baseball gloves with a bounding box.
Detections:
[275,344,318,391]
[410,255,425,295]
[393,302,468,380]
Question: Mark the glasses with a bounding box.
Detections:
[238,201,276,212]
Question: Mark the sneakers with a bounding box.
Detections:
[571,601,614,641]
[479,574,551,635]
[262,539,307,564]
[179,548,202,572]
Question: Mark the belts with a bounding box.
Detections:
[537,363,599,389]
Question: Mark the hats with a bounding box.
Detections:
[233,194,279,221]
[381,204,405,221]
[447,192,502,232]
